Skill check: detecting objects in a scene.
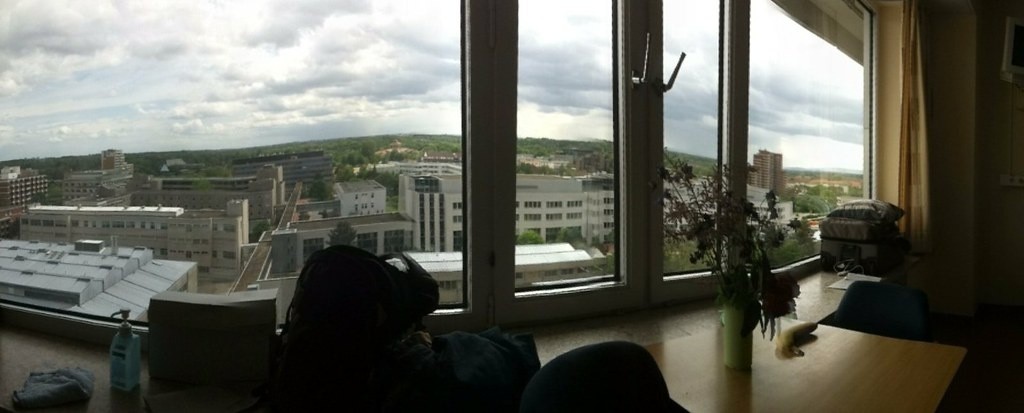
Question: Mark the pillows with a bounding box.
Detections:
[818,217,901,245]
[826,199,905,226]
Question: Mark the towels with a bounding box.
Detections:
[11,367,96,410]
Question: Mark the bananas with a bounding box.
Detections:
[778,321,818,357]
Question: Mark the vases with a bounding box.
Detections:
[716,269,753,370]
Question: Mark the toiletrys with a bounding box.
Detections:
[108,306,141,392]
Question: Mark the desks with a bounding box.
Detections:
[782,271,847,324]
[531,299,969,413]
[0,323,199,413]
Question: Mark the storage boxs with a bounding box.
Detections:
[146,298,277,389]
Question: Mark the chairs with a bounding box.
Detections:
[832,280,931,342]
[519,341,689,413]
